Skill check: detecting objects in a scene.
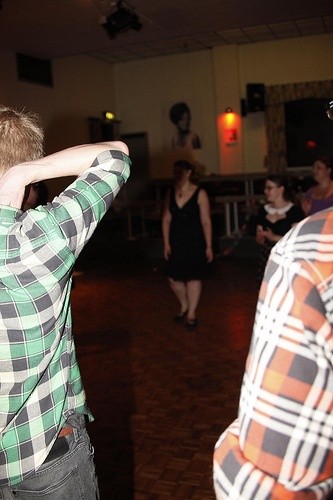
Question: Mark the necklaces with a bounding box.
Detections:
[178,183,189,198]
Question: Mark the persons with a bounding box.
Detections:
[161,159,215,326]
[0,108,133,500]
[255,175,305,294]
[301,160,333,217]
[212,207,333,500]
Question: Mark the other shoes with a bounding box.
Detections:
[174,313,185,324]
[187,319,196,331]
